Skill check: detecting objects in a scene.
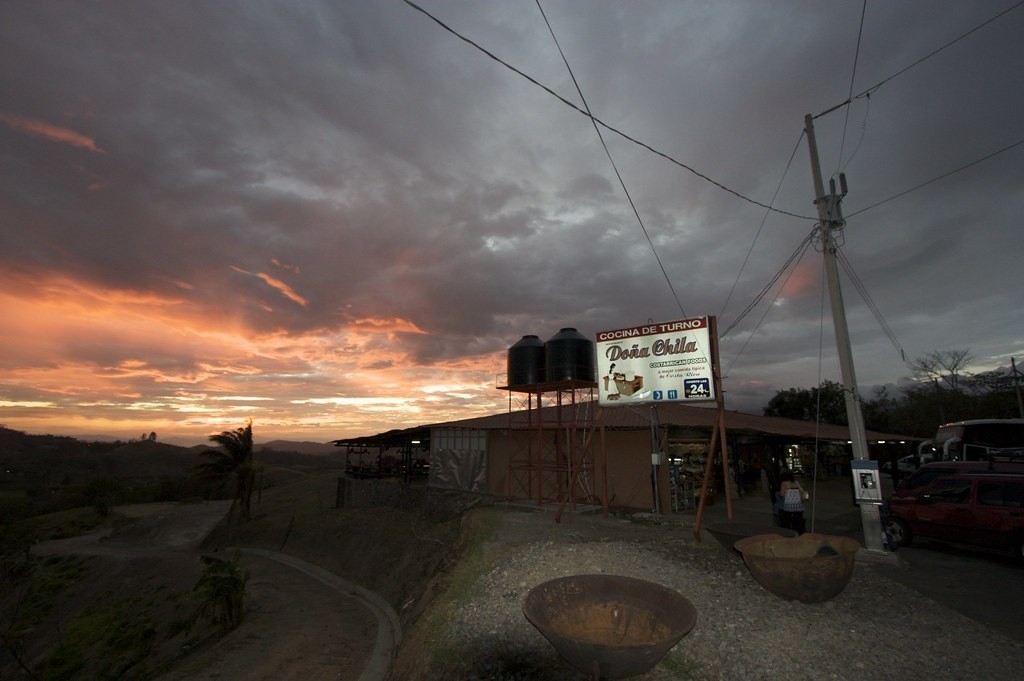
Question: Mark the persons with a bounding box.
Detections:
[879,493,898,552]
[779,470,806,536]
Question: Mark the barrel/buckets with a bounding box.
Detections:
[544,328,595,382]
[508,335,544,386]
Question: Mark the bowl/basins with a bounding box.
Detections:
[705,522,799,555]
[734,532,861,604]
[523,574,698,679]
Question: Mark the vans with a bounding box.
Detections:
[881,462,1024,560]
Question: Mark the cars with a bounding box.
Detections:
[881,454,919,479]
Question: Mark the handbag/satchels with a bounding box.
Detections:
[801,492,809,502]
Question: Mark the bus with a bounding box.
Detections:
[917,417,1024,463]
